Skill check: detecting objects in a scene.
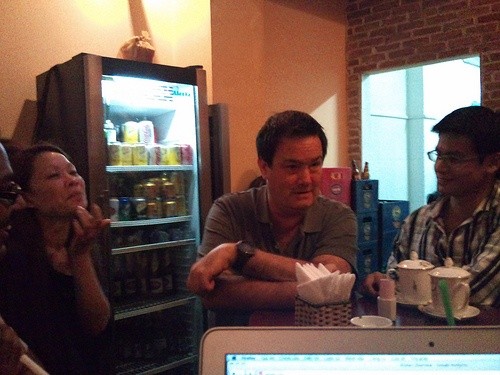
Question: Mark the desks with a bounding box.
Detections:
[248,303,500,329]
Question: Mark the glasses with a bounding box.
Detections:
[427,150,487,165]
[0,181,22,204]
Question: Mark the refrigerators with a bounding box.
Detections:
[35,52,216,375]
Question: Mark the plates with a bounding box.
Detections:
[392,295,432,307]
[417,302,480,320]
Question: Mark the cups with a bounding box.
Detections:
[387,251,435,303]
[426,255,472,316]
[377,296,397,321]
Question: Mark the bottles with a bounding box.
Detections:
[115,307,190,362]
[109,253,176,300]
[352,159,370,189]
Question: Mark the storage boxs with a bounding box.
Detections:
[320,167,409,294]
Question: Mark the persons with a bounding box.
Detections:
[0,143,50,375]
[248,176,266,188]
[359,106,500,309]
[186,110,357,326]
[0,145,114,375]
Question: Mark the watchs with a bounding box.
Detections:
[229,240,255,274]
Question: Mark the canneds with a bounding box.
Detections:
[105,120,192,166]
[109,170,188,220]
